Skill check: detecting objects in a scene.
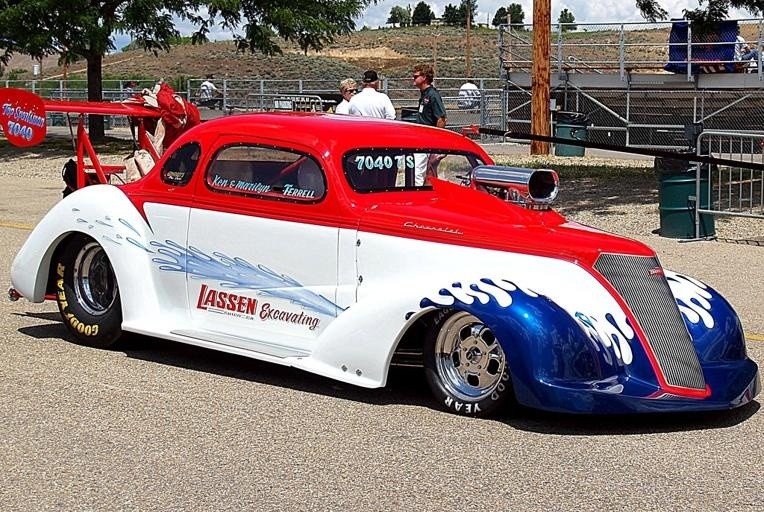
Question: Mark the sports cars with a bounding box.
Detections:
[9,113,760,419]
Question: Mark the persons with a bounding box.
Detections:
[334,78,357,114]
[734,25,764,69]
[347,70,396,120]
[198,74,222,110]
[456,76,485,114]
[412,64,447,187]
[122,81,137,100]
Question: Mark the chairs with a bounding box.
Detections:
[297,160,326,196]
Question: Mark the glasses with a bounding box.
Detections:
[414,75,422,79]
[346,89,358,93]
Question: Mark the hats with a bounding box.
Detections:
[363,70,379,82]
